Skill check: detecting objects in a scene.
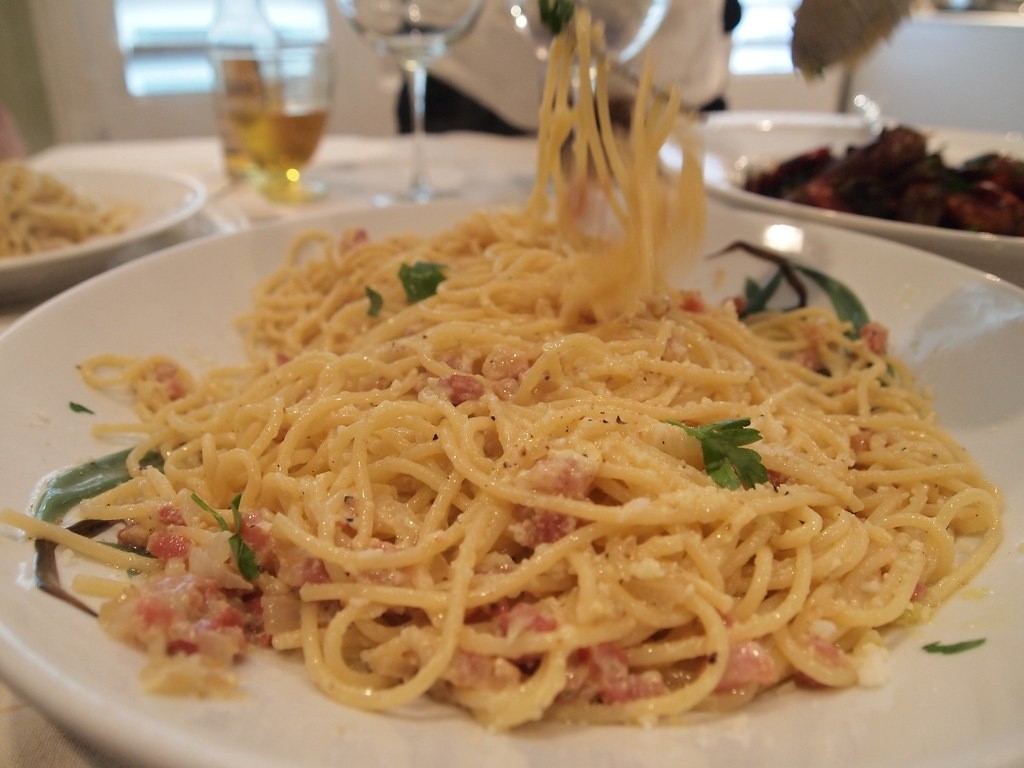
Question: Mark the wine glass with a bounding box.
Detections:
[336,0,483,207]
[255,46,327,205]
[507,0,667,205]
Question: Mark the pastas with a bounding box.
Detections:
[0,8,1002,721]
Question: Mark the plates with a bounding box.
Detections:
[0,165,208,279]
[656,113,1023,242]
[1,204,1023,768]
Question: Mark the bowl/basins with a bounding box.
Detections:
[843,9,1023,129]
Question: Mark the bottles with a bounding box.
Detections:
[208,1,281,187]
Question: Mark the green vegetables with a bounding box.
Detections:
[398,261,447,302]
[658,417,768,490]
[190,491,260,581]
